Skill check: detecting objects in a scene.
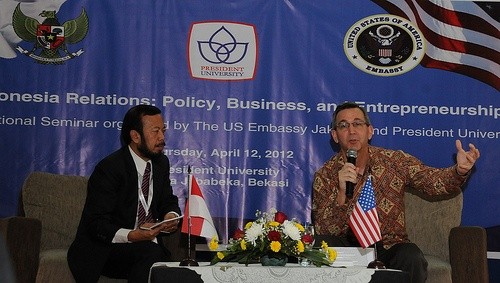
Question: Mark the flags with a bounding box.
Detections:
[348,174,382,248]
[180,174,220,242]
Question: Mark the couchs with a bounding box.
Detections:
[400,186,488,283]
[0,174,184,283]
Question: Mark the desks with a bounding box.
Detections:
[149,260,398,283]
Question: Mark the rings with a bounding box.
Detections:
[171,226,174,229]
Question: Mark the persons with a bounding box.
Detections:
[67,104,184,283]
[311,103,480,283]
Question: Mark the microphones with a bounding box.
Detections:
[345,148,357,200]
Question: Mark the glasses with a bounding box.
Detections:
[335,121,369,128]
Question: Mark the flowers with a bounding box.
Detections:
[208,209,336,270]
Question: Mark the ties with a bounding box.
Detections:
[138,162,156,226]
